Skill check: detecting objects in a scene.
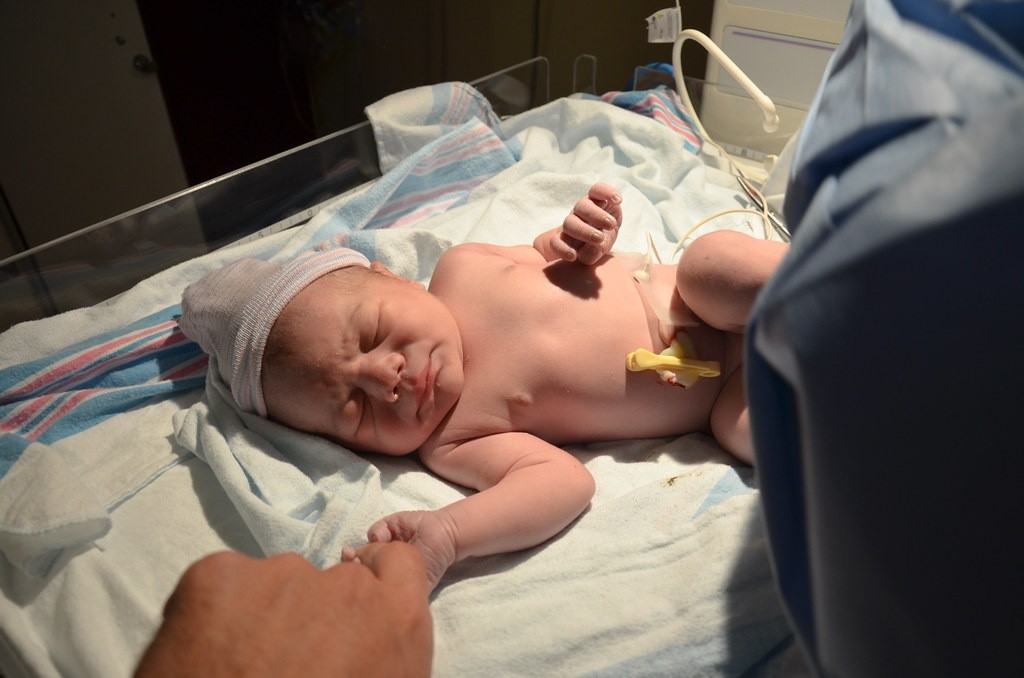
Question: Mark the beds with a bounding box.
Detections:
[0,53,810,678]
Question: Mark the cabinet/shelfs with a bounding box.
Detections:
[699,1,851,158]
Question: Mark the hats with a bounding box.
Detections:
[179,247,371,418]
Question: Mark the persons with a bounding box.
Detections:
[130,541,436,678]
[745,0,1023,678]
[178,182,789,600]
